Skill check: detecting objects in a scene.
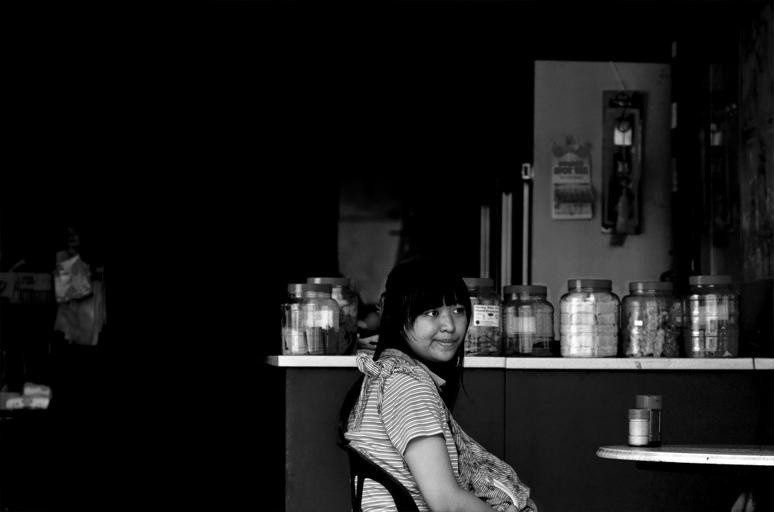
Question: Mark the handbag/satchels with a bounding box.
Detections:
[444,406,532,512]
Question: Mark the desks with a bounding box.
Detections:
[596,441,774,512]
[264,348,774,511]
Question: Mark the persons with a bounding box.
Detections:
[336,246,496,511]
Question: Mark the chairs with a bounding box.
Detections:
[338,442,420,512]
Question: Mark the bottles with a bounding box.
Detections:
[279,285,339,355]
[628,408,651,447]
[305,277,359,354]
[636,394,662,447]
[460,275,741,359]
